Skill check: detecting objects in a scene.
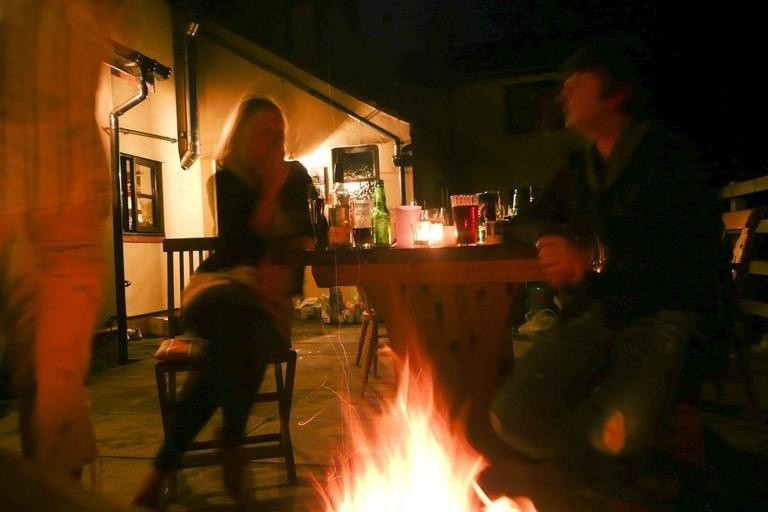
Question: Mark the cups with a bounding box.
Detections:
[453,205,479,245]
[353,201,372,250]
[393,204,420,248]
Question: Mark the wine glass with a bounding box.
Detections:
[305,183,326,254]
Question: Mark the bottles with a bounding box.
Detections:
[496,185,534,222]
[372,179,390,248]
[322,162,351,252]
[440,208,449,226]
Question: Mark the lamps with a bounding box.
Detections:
[182,11,209,43]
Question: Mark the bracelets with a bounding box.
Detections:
[589,234,607,274]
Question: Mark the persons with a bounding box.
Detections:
[488,41,733,498]
[129,95,318,506]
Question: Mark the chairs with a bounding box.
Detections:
[691,209,760,411]
[152,238,297,501]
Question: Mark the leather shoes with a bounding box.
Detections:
[133,469,178,505]
[222,445,244,491]
